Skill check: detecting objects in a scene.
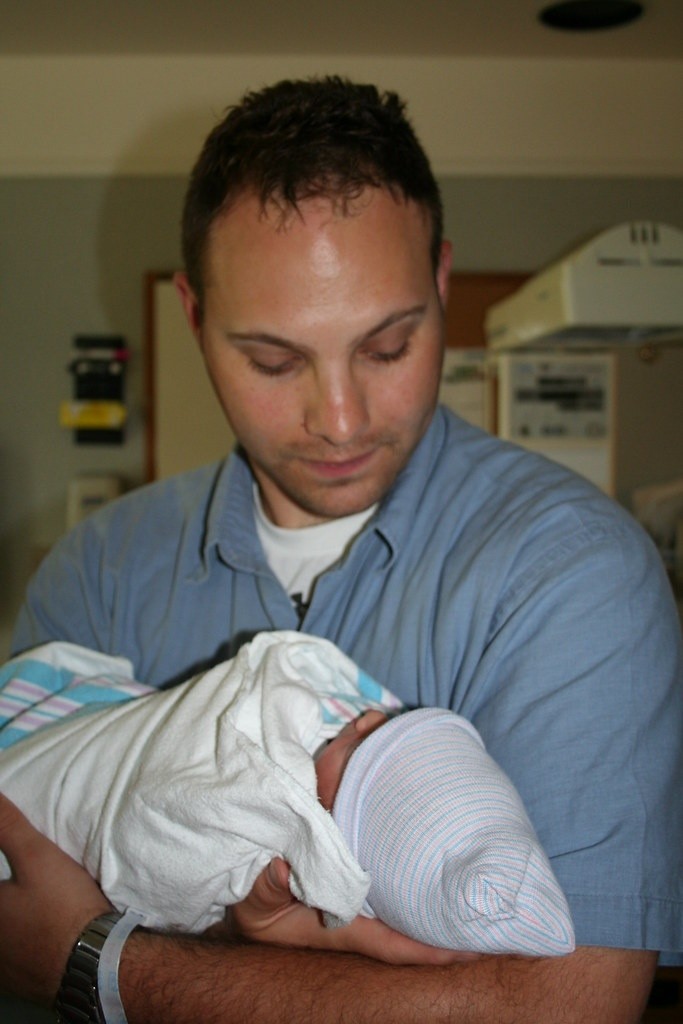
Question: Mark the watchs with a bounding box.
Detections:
[46,911,125,1024]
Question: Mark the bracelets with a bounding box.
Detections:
[101,907,157,1024]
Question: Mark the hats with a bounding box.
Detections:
[330,707,573,958]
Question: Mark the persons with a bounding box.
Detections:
[0,77,683,1024]
[0,630,576,956]
